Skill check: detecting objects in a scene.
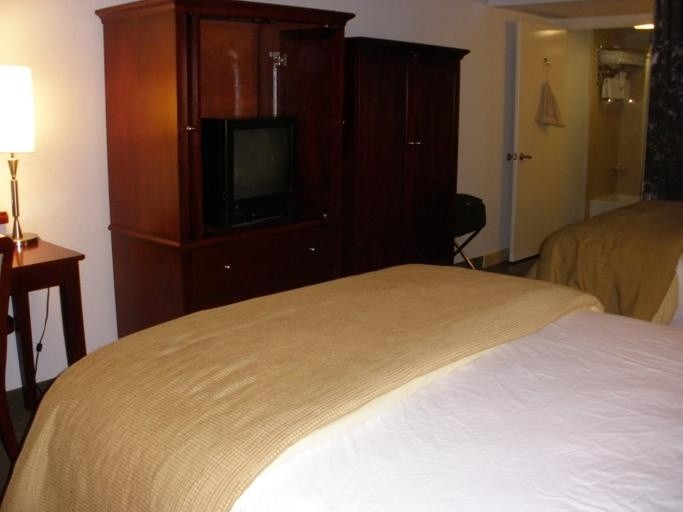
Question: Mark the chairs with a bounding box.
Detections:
[0,238,23,464]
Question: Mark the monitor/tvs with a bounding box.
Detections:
[202,117,302,235]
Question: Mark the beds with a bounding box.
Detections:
[525,200,682,330]
[0,262,683,512]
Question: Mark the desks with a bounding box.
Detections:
[12,237,87,413]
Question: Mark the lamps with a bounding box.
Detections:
[0,64,38,251]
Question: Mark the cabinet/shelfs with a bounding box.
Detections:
[96,0,347,338]
[345,34,470,276]
